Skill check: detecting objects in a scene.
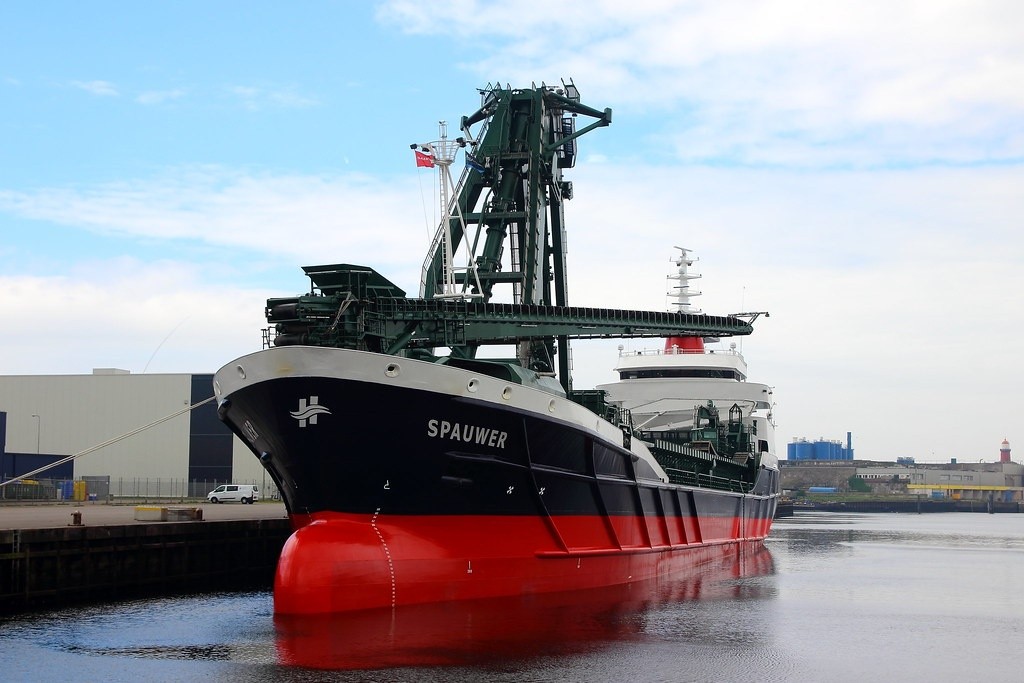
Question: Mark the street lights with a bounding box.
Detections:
[32,413,41,453]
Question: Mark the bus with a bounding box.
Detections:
[928,491,943,499]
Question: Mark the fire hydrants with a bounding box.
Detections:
[68,511,86,526]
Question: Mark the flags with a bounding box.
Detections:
[415,150,434,168]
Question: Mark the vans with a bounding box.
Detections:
[208,486,259,506]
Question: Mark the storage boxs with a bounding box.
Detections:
[136,505,192,521]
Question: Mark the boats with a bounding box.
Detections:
[209,74,782,672]
